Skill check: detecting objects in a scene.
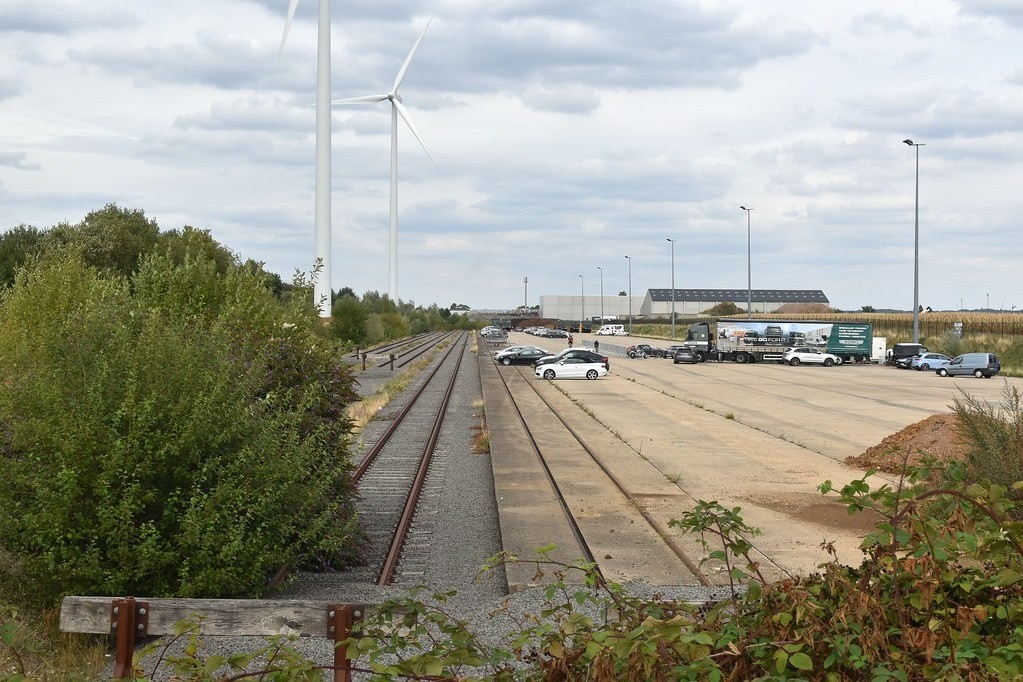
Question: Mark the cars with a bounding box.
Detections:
[534,357,607,381]
[498,349,554,365]
[494,346,542,360]
[896,353,924,369]
[637,345,666,357]
[516,326,570,338]
[481,326,508,339]
[535,349,609,370]
[665,345,684,358]
[525,346,550,353]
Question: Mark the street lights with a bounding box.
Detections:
[597,266,604,325]
[579,275,585,320]
[625,255,633,335]
[903,139,925,344]
[667,238,677,337]
[740,206,754,319]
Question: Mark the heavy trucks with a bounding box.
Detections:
[554,320,592,332]
[685,318,874,364]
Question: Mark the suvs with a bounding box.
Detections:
[935,352,999,379]
[673,347,698,364]
[539,348,597,361]
[911,352,953,372]
[782,346,842,367]
[612,330,630,337]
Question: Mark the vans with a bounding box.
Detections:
[596,326,615,335]
[892,344,929,363]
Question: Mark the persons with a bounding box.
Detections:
[568,335,573,345]
[632,346,642,354]
[594,340,598,348]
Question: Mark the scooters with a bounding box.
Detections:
[630,350,636,359]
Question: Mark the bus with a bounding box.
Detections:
[603,325,624,332]
